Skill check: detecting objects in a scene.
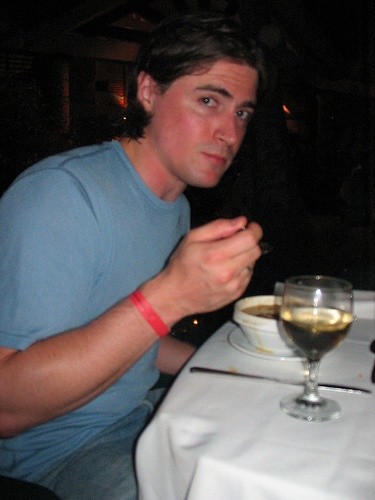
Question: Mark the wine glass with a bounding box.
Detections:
[282,277,355,420]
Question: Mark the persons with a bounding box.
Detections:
[0,18,263,500]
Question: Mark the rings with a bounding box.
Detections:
[245,265,254,273]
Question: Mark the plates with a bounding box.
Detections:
[229,328,309,362]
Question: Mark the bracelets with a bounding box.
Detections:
[129,290,171,338]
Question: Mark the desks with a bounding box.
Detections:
[134,319,375,500]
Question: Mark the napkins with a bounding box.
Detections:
[275,282,375,319]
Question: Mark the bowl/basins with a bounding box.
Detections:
[234,296,320,354]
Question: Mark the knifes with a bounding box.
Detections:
[189,367,373,398]
